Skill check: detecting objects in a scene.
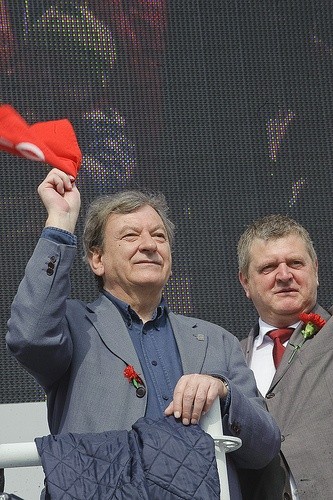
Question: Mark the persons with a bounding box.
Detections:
[223,213,333,500]
[2,165,286,500]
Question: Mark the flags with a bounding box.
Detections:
[1,101,83,185]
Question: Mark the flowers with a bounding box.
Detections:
[298,312,327,339]
[123,365,143,389]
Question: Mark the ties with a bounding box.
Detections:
[266,327,294,371]
